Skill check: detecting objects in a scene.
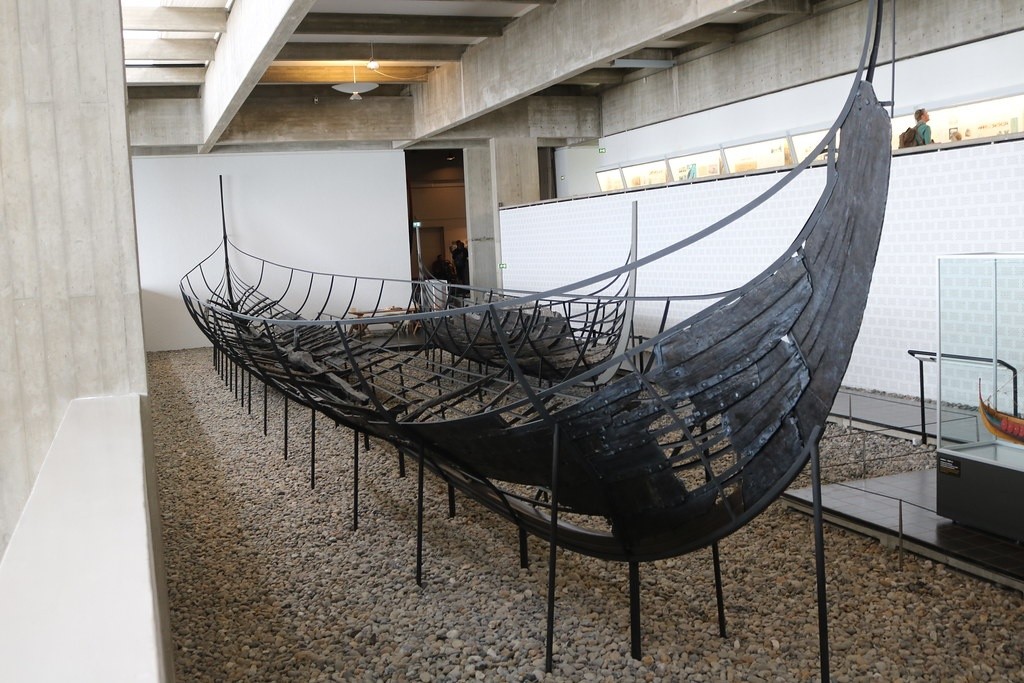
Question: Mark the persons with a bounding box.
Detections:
[950,132,962,142]
[911,108,934,147]
[452,240,468,284]
[432,254,452,283]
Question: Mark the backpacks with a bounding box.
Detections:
[898,124,925,149]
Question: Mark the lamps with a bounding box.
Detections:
[367,42,379,69]
[332,66,378,100]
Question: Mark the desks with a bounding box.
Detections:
[348,308,415,337]
